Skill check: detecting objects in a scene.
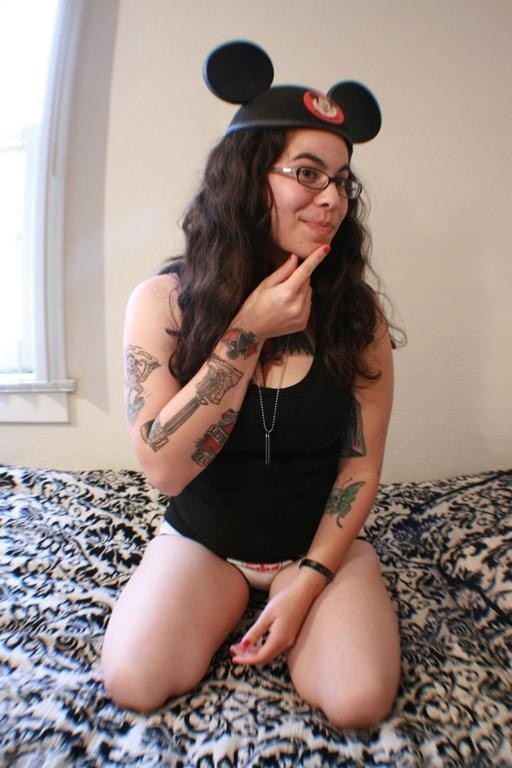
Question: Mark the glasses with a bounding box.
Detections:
[270,166,362,200]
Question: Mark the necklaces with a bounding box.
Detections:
[252,334,294,467]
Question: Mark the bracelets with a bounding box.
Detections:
[298,559,335,583]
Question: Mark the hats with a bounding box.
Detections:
[203,40,381,154]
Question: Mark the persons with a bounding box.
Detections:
[94,34,406,731]
